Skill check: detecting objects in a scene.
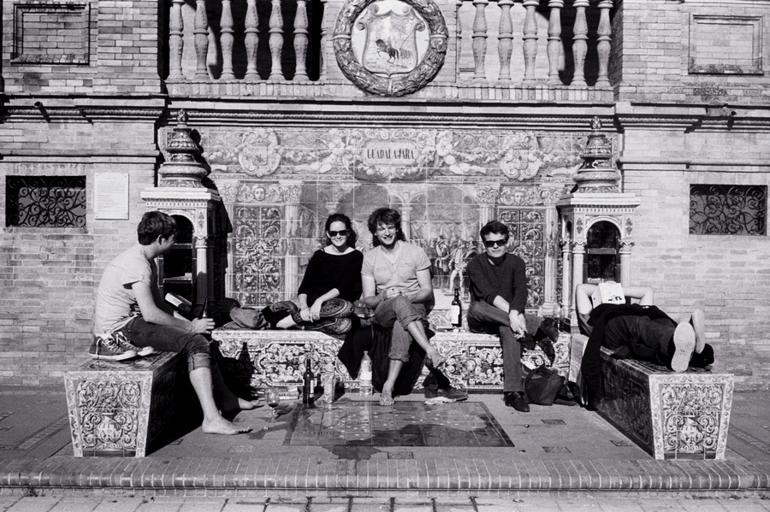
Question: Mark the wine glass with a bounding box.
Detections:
[266,389,281,416]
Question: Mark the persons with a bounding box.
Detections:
[464,219,537,413]
[272,213,364,337]
[359,206,447,408]
[411,233,481,300]
[575,281,715,372]
[91,211,261,437]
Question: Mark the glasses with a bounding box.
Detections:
[327,229,350,237]
[483,238,507,247]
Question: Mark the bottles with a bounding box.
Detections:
[450,287,463,327]
[308,372,325,401]
[302,357,317,409]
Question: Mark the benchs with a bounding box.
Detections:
[210,308,570,404]
[62,348,185,461]
[569,325,737,462]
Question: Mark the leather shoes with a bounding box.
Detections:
[504,390,531,412]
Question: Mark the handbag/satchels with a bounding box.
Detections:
[525,367,566,407]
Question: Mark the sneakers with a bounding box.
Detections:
[671,319,697,373]
[87,335,136,362]
[425,382,470,407]
[686,308,709,356]
[105,331,155,357]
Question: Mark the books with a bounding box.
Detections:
[590,283,627,310]
